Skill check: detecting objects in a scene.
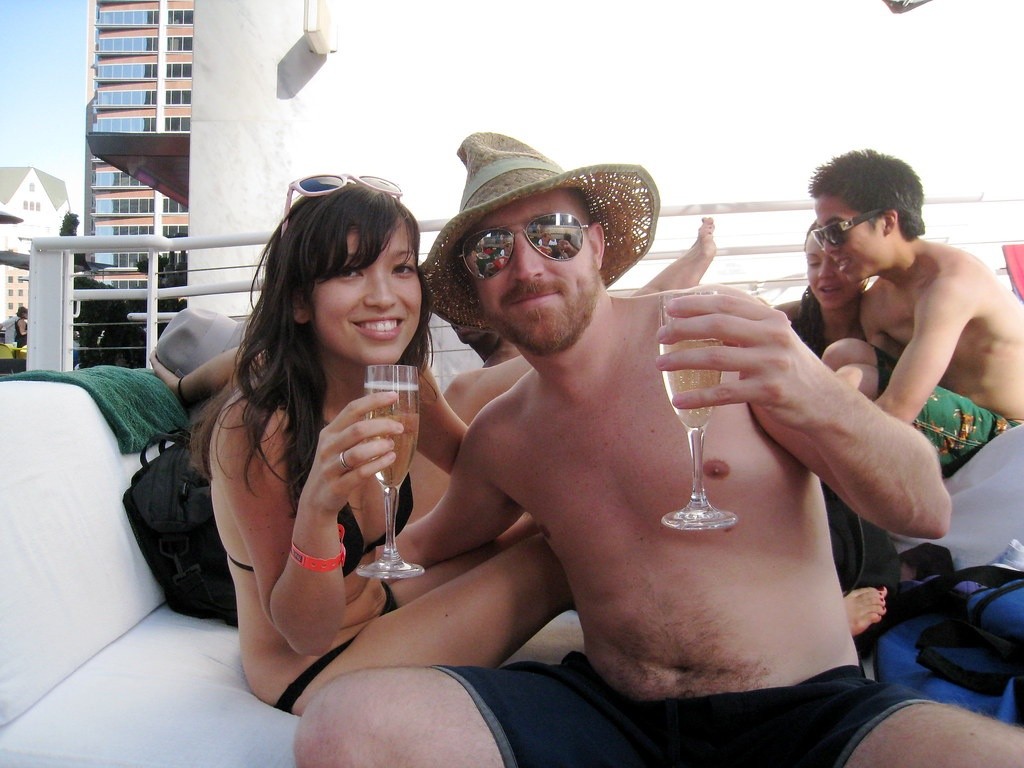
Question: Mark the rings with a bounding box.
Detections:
[338,452,353,471]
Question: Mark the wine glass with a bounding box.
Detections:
[355,365,426,580]
[659,290,738,531]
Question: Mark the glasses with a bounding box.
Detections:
[458,212,590,281]
[281,174,402,238]
[811,206,888,251]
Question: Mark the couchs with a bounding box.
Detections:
[0,365,304,768]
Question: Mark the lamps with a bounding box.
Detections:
[303,0,338,54]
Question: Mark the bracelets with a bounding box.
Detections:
[289,524,348,571]
[178,377,185,396]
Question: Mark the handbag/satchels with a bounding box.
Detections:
[123,429,238,627]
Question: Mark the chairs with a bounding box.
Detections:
[999,244,1024,303]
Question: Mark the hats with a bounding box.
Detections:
[419,133,661,332]
[156,307,250,378]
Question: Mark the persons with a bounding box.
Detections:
[500,233,504,248]
[808,149,1024,476]
[559,240,578,258]
[148,174,889,717]
[537,223,542,237]
[538,231,555,248]
[479,239,484,253]
[164,262,186,312]
[292,135,1024,768]
[502,241,512,257]
[770,216,867,360]
[0,306,28,348]
